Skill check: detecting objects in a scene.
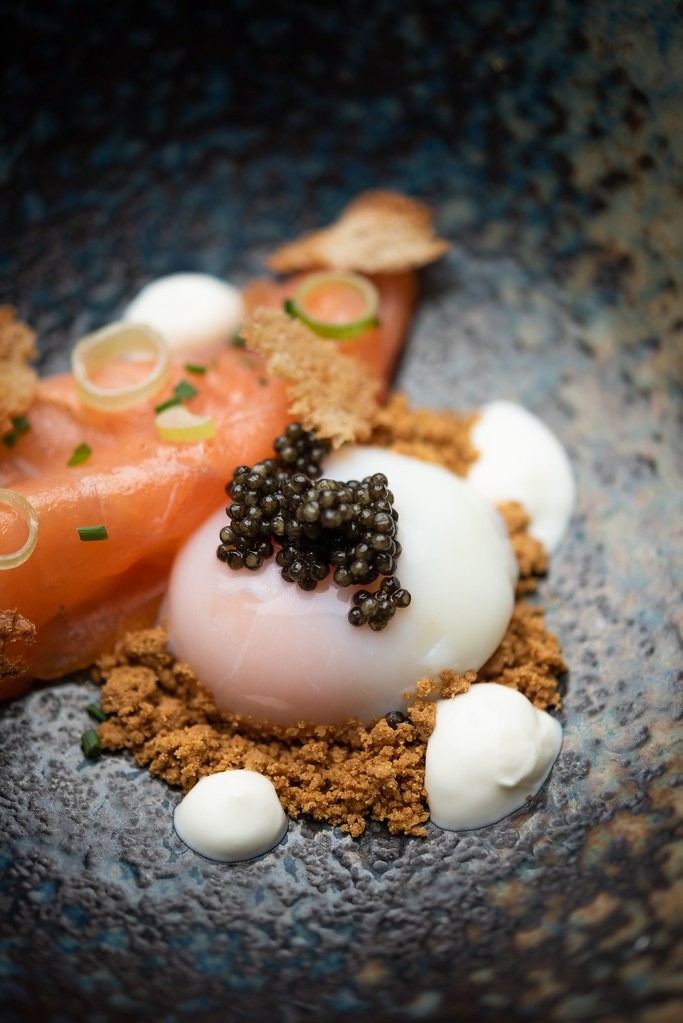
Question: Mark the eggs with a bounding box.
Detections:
[162,443,518,740]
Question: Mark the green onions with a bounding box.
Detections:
[0,273,385,757]
